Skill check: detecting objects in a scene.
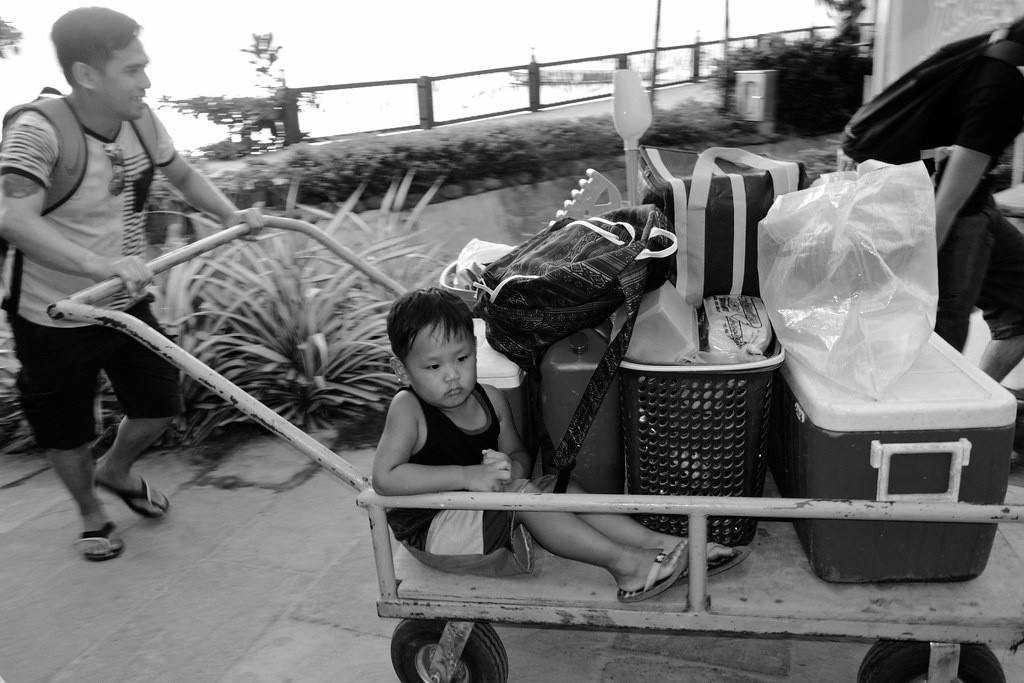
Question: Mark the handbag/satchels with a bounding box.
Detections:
[635,145,807,310]
[757,158,939,402]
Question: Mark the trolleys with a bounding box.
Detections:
[45,215,1023,683]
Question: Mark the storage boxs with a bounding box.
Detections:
[472,318,529,444]
[769,330,1017,586]
[619,344,785,574]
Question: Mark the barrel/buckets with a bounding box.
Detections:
[535,319,624,494]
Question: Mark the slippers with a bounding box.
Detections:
[616,540,690,602]
[78,520,123,562]
[671,546,752,587]
[96,473,168,522]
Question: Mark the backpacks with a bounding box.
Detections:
[468,204,677,493]
[841,30,1002,164]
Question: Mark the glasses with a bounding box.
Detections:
[103,141,125,197]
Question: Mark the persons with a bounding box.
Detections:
[0,7,267,562]
[373,288,752,603]
[919,16,1024,384]
